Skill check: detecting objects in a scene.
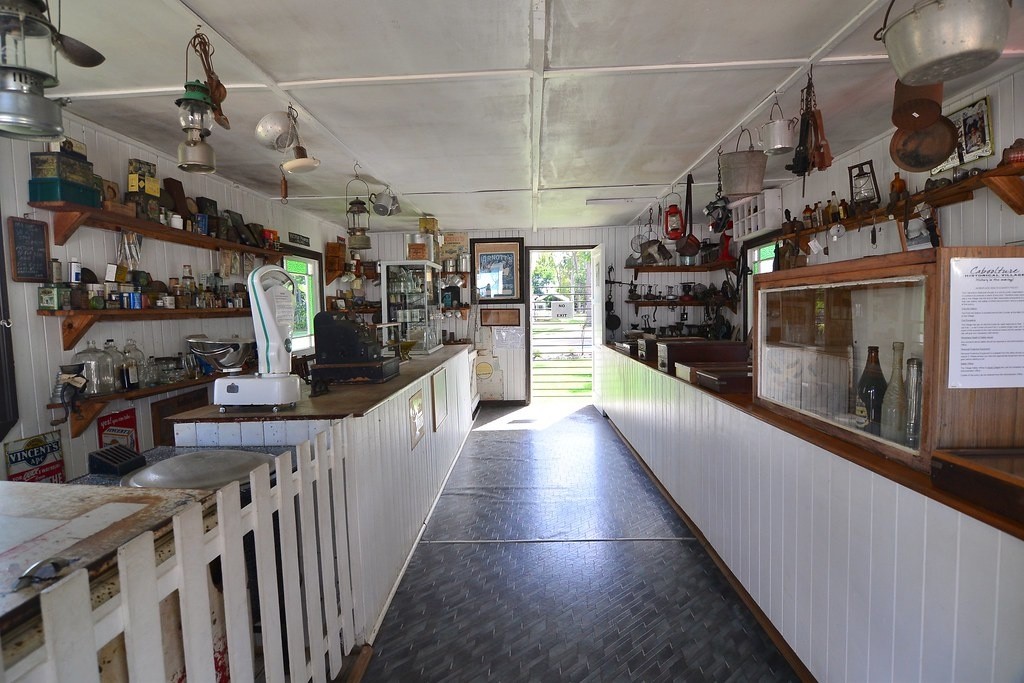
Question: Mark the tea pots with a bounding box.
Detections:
[368,193,399,216]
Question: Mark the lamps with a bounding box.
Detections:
[663,193,684,240]
[847,159,881,214]
[0,0,65,142]
[175,24,216,175]
[345,164,372,250]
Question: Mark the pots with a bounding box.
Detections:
[443,251,471,273]
[407,234,434,263]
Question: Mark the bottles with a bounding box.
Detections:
[51,257,249,309]
[74,338,206,396]
[881,341,907,443]
[802,192,846,229]
[856,346,889,437]
[890,172,906,201]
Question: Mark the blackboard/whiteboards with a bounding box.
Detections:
[7,216,51,282]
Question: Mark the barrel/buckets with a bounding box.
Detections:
[717,128,768,196]
[640,230,659,261]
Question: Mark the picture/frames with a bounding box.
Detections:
[896,207,944,252]
[469,236,524,305]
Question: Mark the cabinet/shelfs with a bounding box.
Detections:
[27,201,290,440]
[733,189,783,242]
[624,262,736,307]
[380,260,444,355]
